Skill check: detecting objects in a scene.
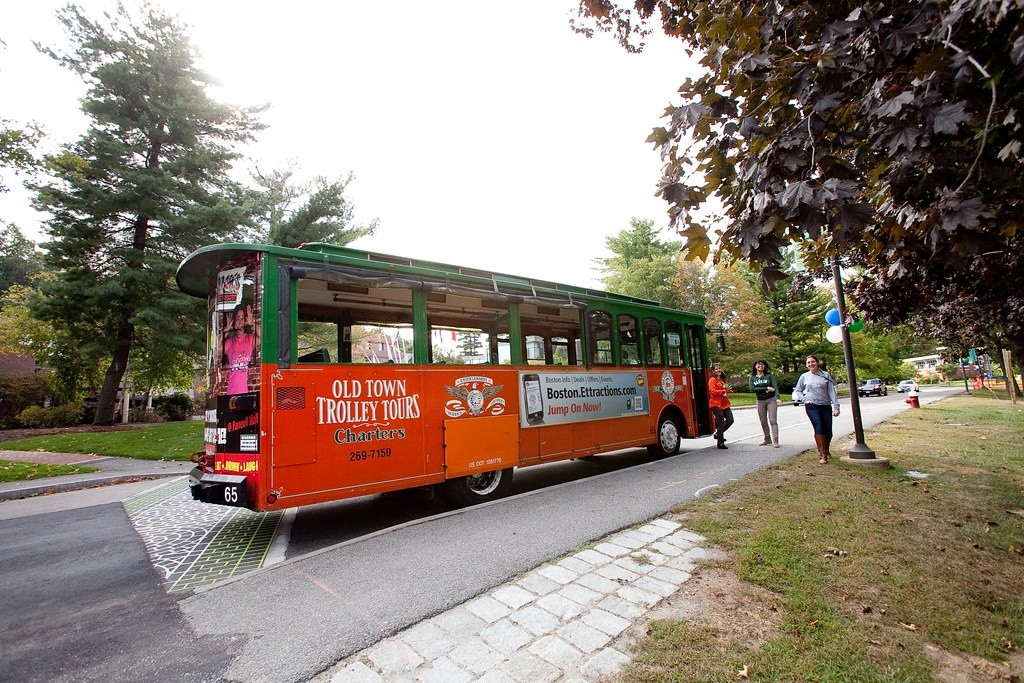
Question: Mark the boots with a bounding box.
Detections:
[814,434,833,464]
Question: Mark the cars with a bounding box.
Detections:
[858,378,887,398]
[897,380,920,393]
[83,396,159,421]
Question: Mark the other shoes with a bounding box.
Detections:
[772,438,780,448]
[759,435,772,446]
[716,438,728,449]
[713,428,727,441]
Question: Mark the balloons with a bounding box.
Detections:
[825,308,840,326]
[849,318,863,333]
[825,325,844,344]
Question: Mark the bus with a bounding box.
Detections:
[176,241,715,515]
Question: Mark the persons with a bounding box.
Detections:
[748,360,781,448]
[217,305,262,396]
[622,349,629,366]
[708,363,734,450]
[793,355,840,465]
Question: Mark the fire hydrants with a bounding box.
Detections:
[905,391,919,409]
